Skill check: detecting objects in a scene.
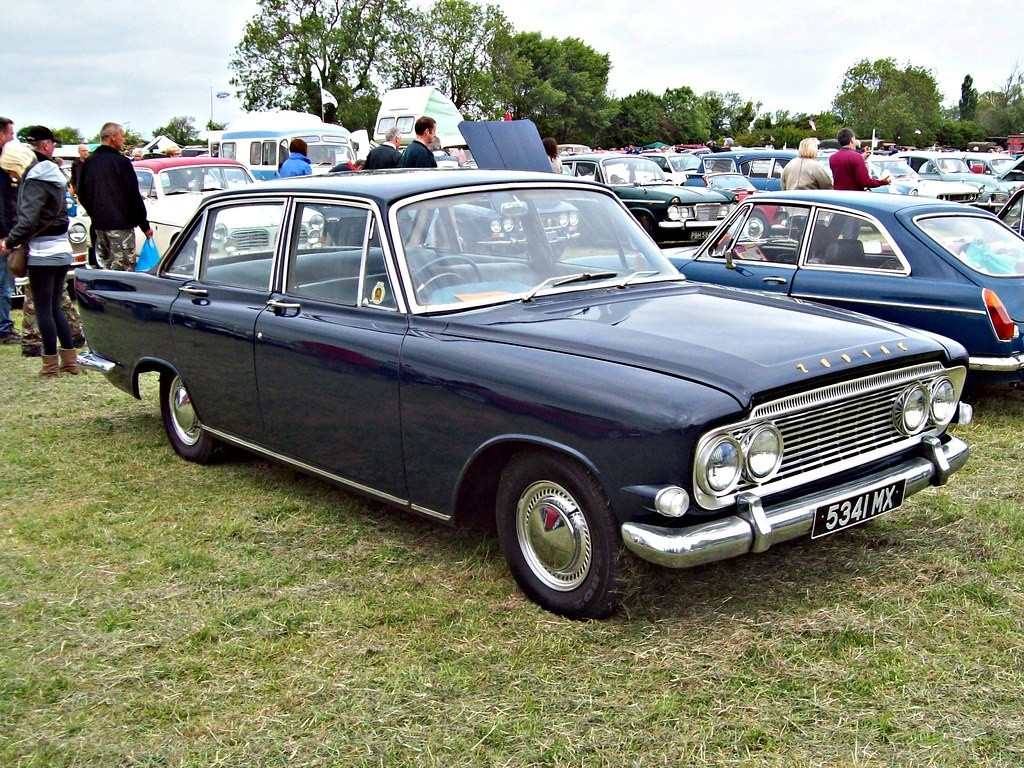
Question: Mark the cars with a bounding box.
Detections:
[644,148,917,200]
[559,188,1024,394]
[76,159,326,271]
[868,155,984,206]
[11,217,91,301]
[66,167,972,621]
[559,154,738,251]
[317,119,579,262]
[890,151,1024,216]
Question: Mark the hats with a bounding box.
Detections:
[723,138,734,145]
[26,125,63,144]
[662,146,667,149]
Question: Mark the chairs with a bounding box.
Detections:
[427,261,531,289]
[822,239,866,266]
[289,271,399,310]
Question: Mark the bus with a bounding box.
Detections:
[181,149,207,158]
[208,112,357,185]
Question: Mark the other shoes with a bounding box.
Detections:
[72,335,86,349]
[21,346,61,356]
[0,326,22,344]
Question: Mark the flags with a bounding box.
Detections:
[321,86,338,108]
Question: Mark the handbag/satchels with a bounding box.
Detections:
[135,235,161,272]
[7,238,28,277]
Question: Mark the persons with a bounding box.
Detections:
[991,147,1002,153]
[404,117,450,168]
[829,128,891,240]
[706,138,734,173]
[861,145,905,156]
[0,117,219,378]
[363,127,404,170]
[281,139,313,178]
[542,138,563,174]
[781,138,833,226]
[626,142,640,155]
[329,159,364,173]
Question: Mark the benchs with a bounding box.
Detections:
[200,247,385,290]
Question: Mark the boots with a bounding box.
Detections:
[59,348,80,374]
[36,355,60,377]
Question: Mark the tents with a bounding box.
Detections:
[643,142,671,150]
